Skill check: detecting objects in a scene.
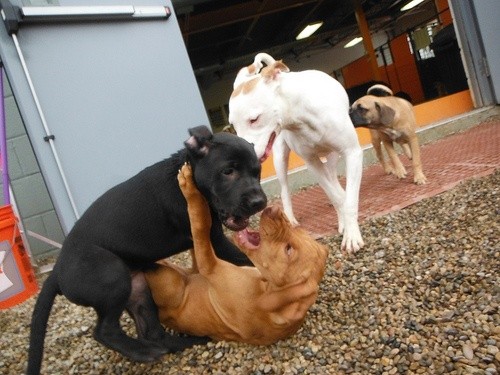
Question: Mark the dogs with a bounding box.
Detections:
[349,84,429,186]
[23,125,329,375]
[227,53,366,255]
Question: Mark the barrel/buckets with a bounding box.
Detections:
[0,205,40,310]
